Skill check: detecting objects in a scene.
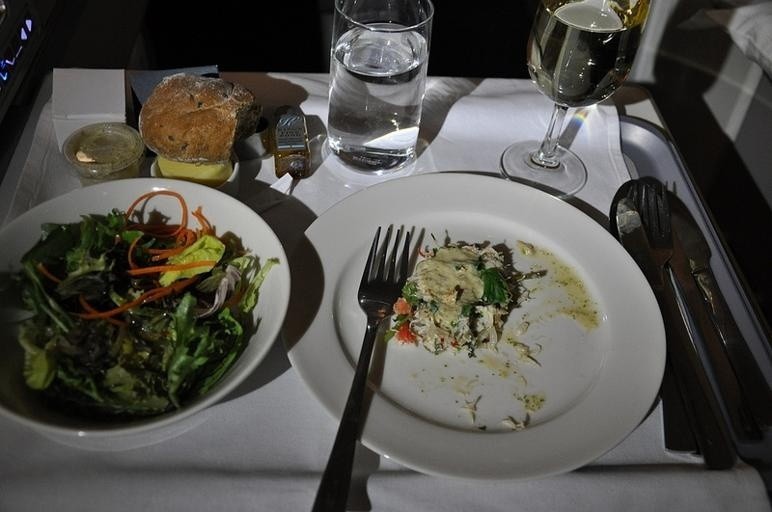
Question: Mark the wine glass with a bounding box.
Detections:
[501,0,652,200]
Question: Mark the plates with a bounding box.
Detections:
[1,176,292,440]
[281,172,667,485]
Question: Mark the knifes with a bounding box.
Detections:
[616,197,734,469]
[666,189,771,429]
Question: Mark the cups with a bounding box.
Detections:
[150,148,241,198]
[328,0,434,175]
[62,122,147,188]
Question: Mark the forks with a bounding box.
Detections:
[310,223,411,512]
[629,184,771,470]
